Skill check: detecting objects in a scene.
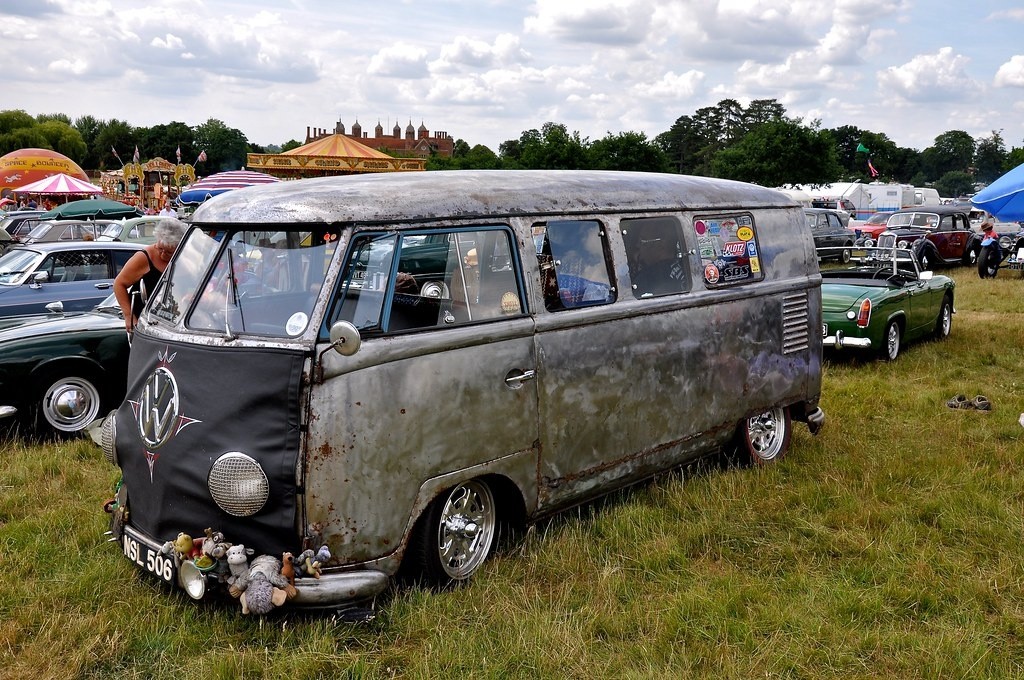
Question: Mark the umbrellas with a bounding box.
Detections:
[0,198,18,208]
[968,162,1024,223]
[38,196,144,237]
[175,167,288,259]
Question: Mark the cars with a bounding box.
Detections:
[850,211,904,250]
[803,207,857,265]
[374,230,476,303]
[0,283,173,442]
[865,206,977,271]
[0,209,180,247]
[0,241,158,331]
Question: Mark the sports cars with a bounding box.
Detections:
[804,247,957,363]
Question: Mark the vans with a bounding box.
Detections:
[767,180,942,215]
[102,169,827,611]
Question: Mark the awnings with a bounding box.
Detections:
[11,173,103,196]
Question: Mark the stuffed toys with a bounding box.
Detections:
[156,527,331,615]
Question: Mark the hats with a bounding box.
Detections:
[978,222,993,232]
[465,248,480,266]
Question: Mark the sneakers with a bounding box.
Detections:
[972,395,991,414]
[945,395,973,410]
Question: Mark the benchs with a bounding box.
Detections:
[308,290,483,327]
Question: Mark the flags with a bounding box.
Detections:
[176,147,181,162]
[198,152,207,162]
[133,147,139,164]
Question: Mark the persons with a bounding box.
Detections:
[241,237,279,292]
[981,221,1000,275]
[113,219,188,333]
[159,207,178,219]
[0,194,37,211]
[162,190,169,210]
